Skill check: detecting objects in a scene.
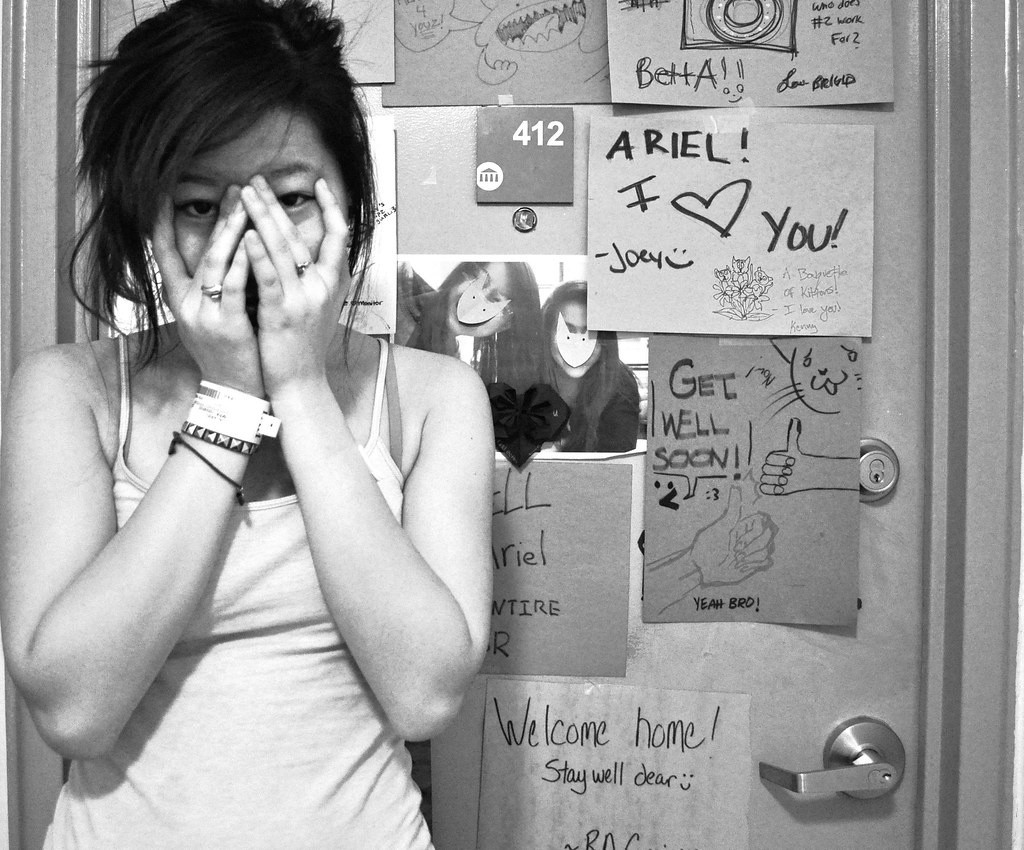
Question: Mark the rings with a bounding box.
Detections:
[201,285,222,299]
[296,262,311,276]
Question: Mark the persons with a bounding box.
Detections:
[533,279,640,453]
[0,0,494,849]
[395,261,547,398]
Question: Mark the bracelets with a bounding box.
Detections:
[168,379,283,506]
